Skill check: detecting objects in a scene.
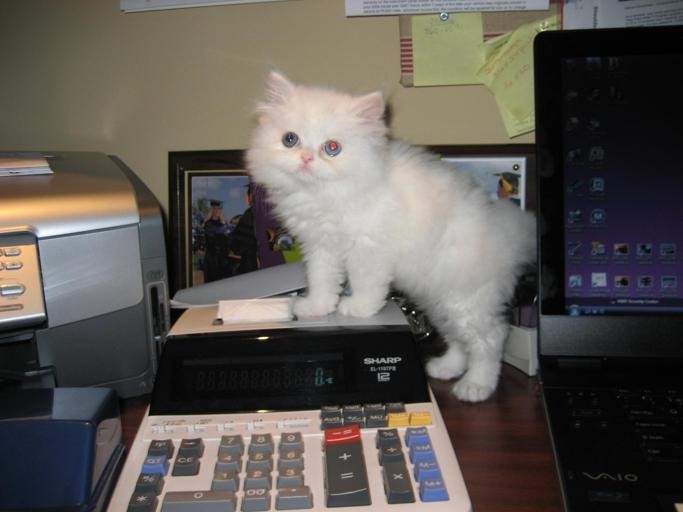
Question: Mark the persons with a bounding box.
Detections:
[224,185,257,273]
[199,199,232,283]
[493,172,520,207]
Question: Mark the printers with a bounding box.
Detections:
[0,152,179,403]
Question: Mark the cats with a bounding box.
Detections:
[243,70,537,403]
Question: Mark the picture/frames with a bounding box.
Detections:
[168,143,539,330]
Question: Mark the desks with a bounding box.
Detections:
[120,354,568,512]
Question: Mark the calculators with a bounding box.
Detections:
[104,295,474,512]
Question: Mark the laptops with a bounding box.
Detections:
[532,26,682,512]
